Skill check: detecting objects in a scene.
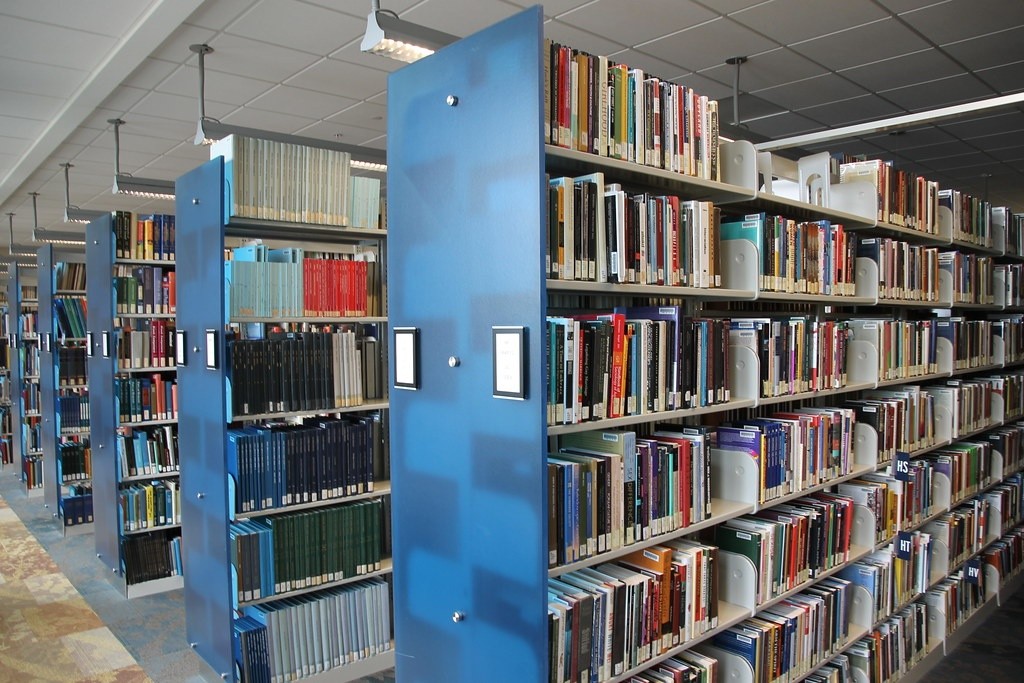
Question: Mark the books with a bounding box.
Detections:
[109,209,183,586]
[0,291,14,464]
[544,39,1024,683]
[51,262,94,527]
[19,285,45,490]
[224,134,389,683]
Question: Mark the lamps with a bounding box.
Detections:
[360,0,815,162]
[188,44,387,174]
[59,163,111,224]
[28,192,85,245]
[107,118,176,199]
[5,213,40,257]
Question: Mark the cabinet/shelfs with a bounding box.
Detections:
[0,262,44,499]
[175,155,396,683]
[388,6,1024,683]
[35,244,94,537]
[86,212,184,599]
